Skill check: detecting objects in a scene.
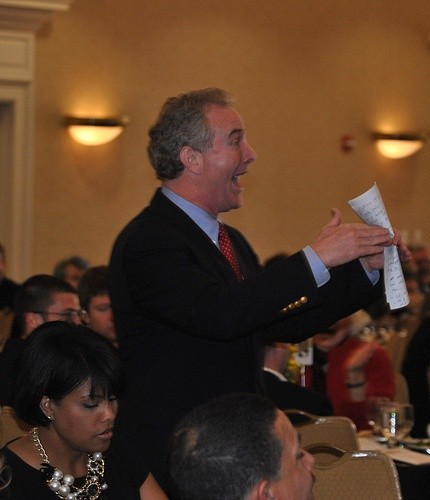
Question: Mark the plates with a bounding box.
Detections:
[400,439,430,452]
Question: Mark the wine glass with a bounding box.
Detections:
[376,404,414,452]
[363,397,391,439]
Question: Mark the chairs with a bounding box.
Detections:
[281,409,403,500]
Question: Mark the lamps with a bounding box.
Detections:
[370,131,427,160]
[50,114,131,147]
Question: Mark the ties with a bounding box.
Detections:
[218,222,243,282]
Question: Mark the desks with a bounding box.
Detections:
[357,437,430,467]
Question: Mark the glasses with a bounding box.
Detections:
[36,307,91,322]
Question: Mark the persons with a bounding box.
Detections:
[0,245,430,500]
[107,88,411,473]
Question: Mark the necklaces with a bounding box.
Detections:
[28,426,109,500]
